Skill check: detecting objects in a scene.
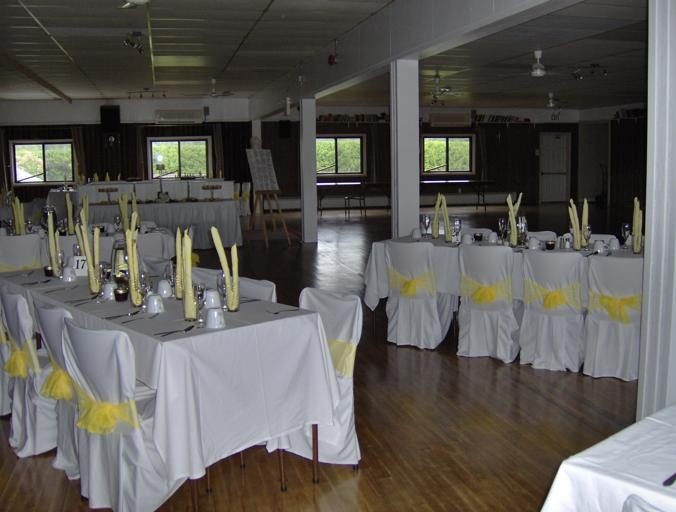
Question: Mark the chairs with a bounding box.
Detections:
[238,288,364,492]
[2,287,58,459]
[586,254,643,380]
[63,319,202,510]
[586,235,618,248]
[189,268,224,293]
[518,250,591,374]
[342,176,367,220]
[0,303,12,418]
[33,305,155,481]
[462,228,493,241]
[529,231,558,244]
[137,257,175,281]
[457,244,518,364]
[239,277,278,301]
[387,241,459,350]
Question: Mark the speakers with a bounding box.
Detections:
[100,105,121,143]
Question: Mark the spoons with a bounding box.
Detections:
[153,325,194,337]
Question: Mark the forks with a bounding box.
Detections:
[264,308,301,315]
[120,312,160,325]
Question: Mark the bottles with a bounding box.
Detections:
[560,237,571,248]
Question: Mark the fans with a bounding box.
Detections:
[501,48,566,82]
[543,91,558,109]
[420,74,453,97]
[181,77,235,99]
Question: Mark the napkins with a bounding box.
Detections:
[81,227,100,290]
[125,228,143,306]
[183,227,198,320]
[440,194,453,242]
[432,192,441,235]
[568,197,582,248]
[175,225,182,298]
[577,198,591,241]
[505,192,522,246]
[209,225,240,308]
[48,211,63,278]
[75,211,86,257]
[632,198,642,250]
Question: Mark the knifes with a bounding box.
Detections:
[63,295,111,307]
[103,310,140,321]
[20,278,51,286]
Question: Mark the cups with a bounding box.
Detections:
[572,230,581,250]
[62,266,76,282]
[205,307,227,329]
[57,222,75,236]
[7,219,33,236]
[411,228,422,241]
[175,270,195,322]
[87,269,130,302]
[444,225,452,242]
[431,222,439,239]
[593,237,620,253]
[73,243,82,256]
[528,236,538,250]
[225,281,240,311]
[113,216,130,233]
[146,263,177,314]
[204,288,220,309]
[43,251,64,277]
[544,240,556,250]
[631,232,642,254]
[460,232,498,244]
[509,228,517,248]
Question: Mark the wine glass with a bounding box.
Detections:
[582,223,592,251]
[193,282,207,332]
[498,216,507,247]
[216,270,234,312]
[452,218,462,245]
[422,213,432,239]
[621,223,630,250]
[518,215,525,248]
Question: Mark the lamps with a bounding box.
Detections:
[121,30,150,58]
[572,62,609,82]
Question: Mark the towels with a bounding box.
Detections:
[427,191,650,256]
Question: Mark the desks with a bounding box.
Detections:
[313,180,366,217]
[0,176,253,252]
[536,401,676,512]
[417,178,531,210]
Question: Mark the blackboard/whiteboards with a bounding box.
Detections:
[245,149,281,191]
